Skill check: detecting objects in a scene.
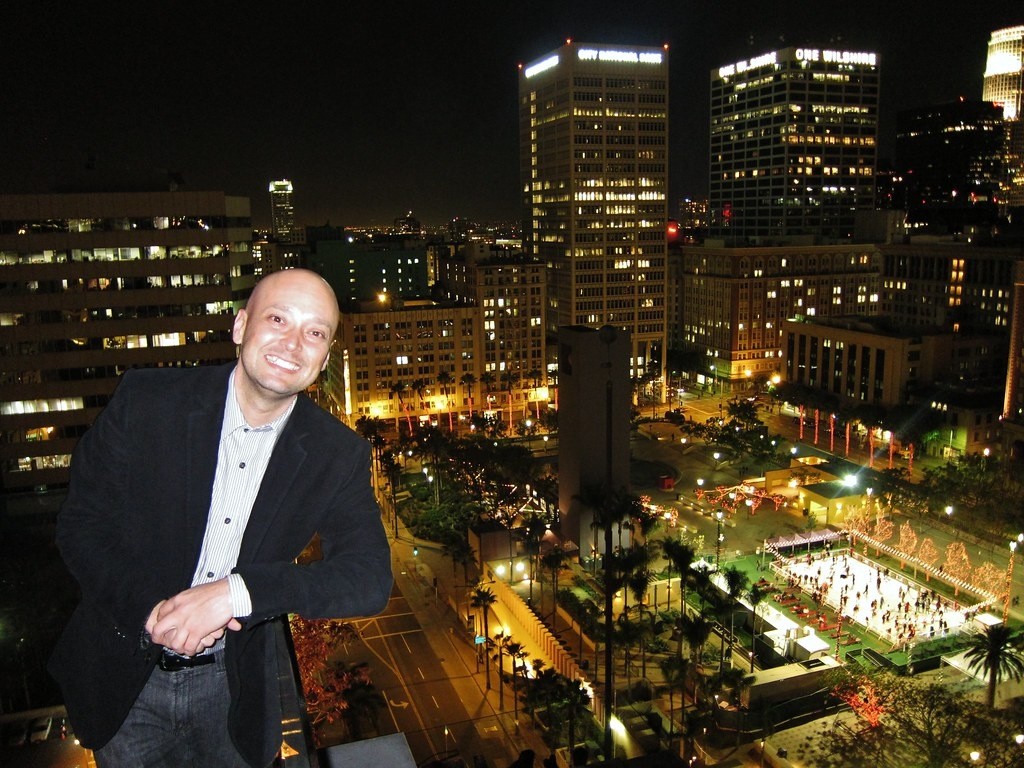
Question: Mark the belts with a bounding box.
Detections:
[156,649,215,674]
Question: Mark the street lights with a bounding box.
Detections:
[374,466,405,538]
[455,579,498,668]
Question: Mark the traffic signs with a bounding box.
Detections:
[474,636,486,645]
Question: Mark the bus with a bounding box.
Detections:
[888,437,915,460]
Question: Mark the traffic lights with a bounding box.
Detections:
[414,548,418,555]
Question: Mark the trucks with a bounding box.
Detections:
[326,731,419,768]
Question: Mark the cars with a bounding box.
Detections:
[676,388,684,393]
[666,391,675,398]
[747,396,760,402]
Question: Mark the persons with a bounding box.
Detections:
[52,267,395,766]
[751,538,982,657]
